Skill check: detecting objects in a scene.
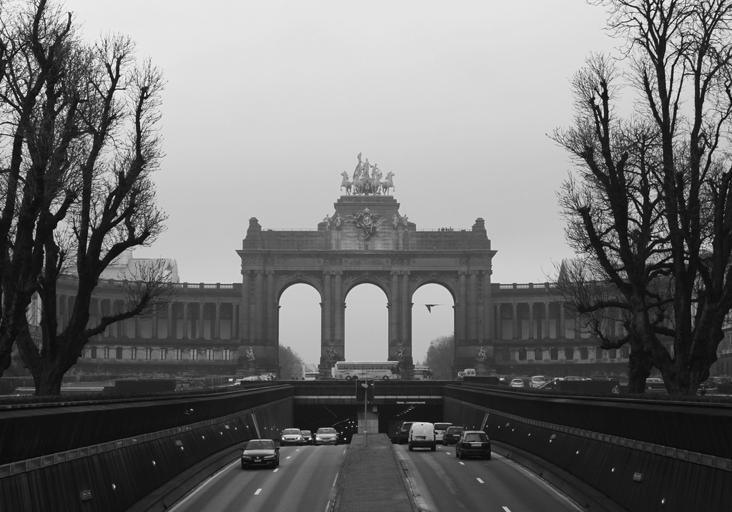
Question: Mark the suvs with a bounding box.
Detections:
[396,420,494,461]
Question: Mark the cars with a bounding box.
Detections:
[509,372,610,389]
[238,437,282,469]
[232,372,276,386]
[279,425,350,446]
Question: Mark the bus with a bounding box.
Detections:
[333,359,405,381]
[303,371,319,381]
[414,368,435,381]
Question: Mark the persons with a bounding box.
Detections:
[372,162,379,175]
[402,213,408,232]
[392,214,399,229]
[360,157,373,174]
[335,213,345,230]
[323,214,334,231]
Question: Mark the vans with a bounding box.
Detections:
[464,367,477,377]
[646,377,662,384]
